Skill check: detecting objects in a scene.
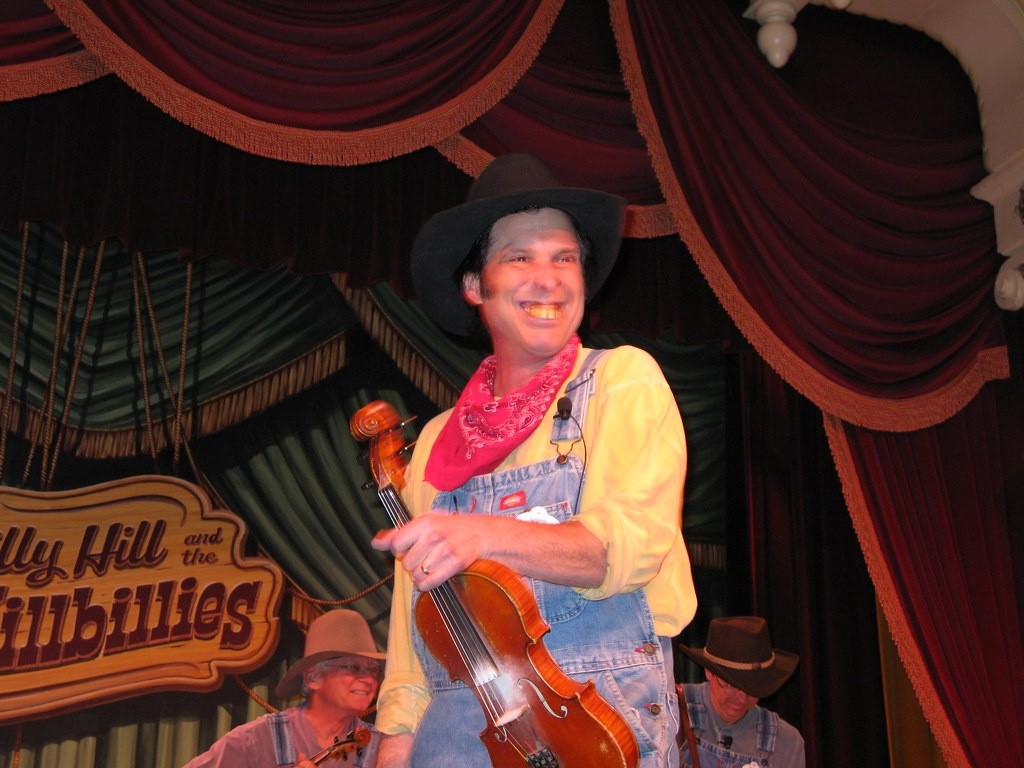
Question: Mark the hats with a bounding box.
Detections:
[677,615,800,699]
[407,153,626,338]
[275,609,386,703]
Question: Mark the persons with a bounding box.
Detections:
[185,609,385,768]
[370,155,698,767]
[679,617,806,768]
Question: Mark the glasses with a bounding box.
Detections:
[328,663,381,676]
[717,677,739,697]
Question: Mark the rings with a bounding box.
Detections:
[421,563,429,575]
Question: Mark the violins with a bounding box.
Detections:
[282,724,373,768]
[347,399,644,768]
